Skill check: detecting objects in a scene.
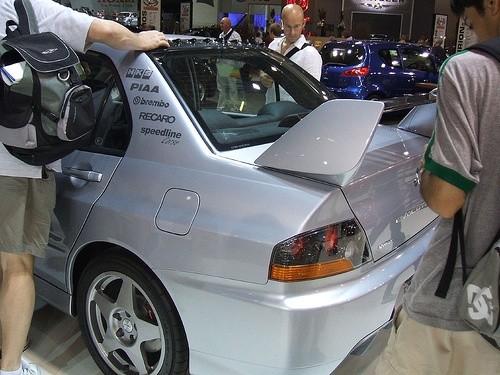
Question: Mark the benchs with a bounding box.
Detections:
[197,101,307,129]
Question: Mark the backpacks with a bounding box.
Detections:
[0,0,96,165]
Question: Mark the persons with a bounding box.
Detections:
[260,4,322,103]
[258,26,262,38]
[342,30,350,39]
[0,0,170,375]
[399,34,407,43]
[268,23,282,50]
[262,32,273,46]
[418,35,425,43]
[215,18,242,111]
[431,36,447,58]
[255,33,263,44]
[374,0,500,375]
[365,150,405,251]
[260,27,267,39]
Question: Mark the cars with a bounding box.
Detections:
[29,36,453,375]
[319,40,444,106]
[112,12,139,27]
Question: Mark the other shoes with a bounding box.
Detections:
[0,358,39,375]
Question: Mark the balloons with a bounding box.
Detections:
[287,0,315,37]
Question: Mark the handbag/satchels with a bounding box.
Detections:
[432,40,500,349]
